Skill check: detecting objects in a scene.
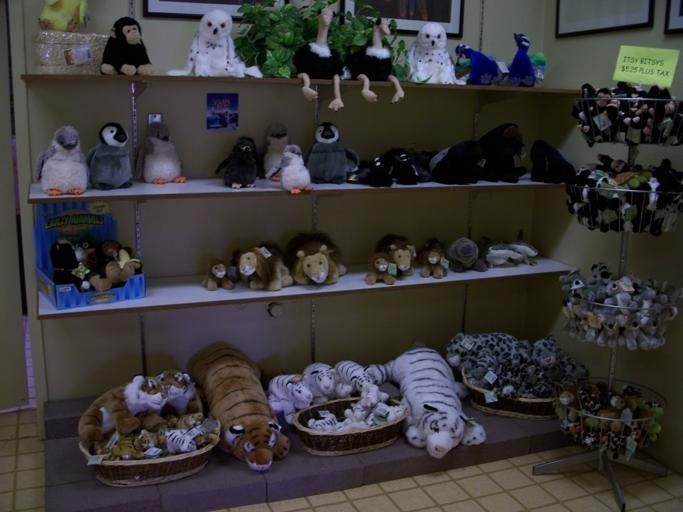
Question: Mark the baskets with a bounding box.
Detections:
[79,443,218,488]
[461,366,557,422]
[293,395,410,456]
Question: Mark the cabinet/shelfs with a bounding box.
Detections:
[21,73,582,512]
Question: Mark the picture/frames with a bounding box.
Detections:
[555,0,683,38]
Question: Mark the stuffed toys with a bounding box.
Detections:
[38,1,91,34]
[80,339,290,472]
[293,6,544,111]
[570,81,683,146]
[565,152,683,236]
[214,121,570,193]
[201,232,345,292]
[100,17,155,77]
[268,339,485,457]
[34,121,187,196]
[558,263,683,350]
[365,228,539,286]
[447,332,663,464]
[166,9,263,79]
[30,0,683,472]
[49,237,143,292]
